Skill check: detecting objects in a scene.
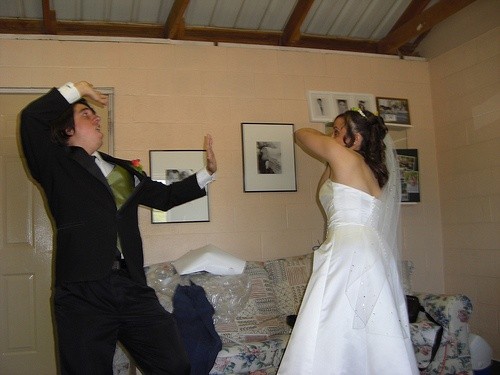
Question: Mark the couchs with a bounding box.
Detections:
[110,252,474,375]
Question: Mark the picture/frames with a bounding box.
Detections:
[307,89,378,123]
[376,96,412,127]
[149,149,210,224]
[241,122,298,193]
[392,148,422,206]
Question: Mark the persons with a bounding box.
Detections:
[338,100,347,114]
[317,99,326,115]
[276,110,421,375]
[19,81,217,375]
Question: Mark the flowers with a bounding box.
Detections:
[130,159,143,174]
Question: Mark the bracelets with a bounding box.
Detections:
[207,167,214,173]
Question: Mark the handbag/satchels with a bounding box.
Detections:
[405,294,444,373]
